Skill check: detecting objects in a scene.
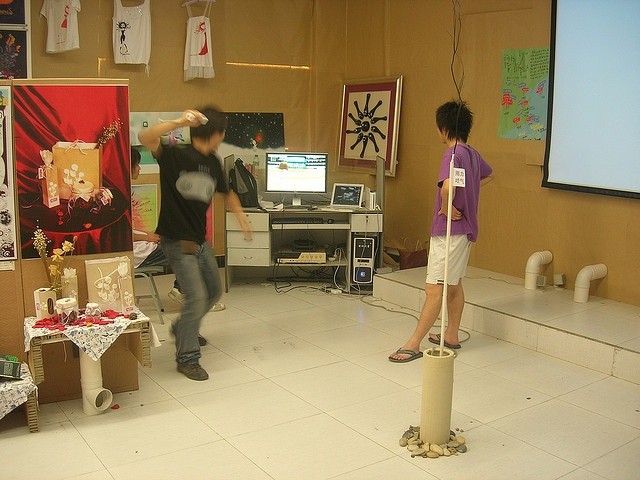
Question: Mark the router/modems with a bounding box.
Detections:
[294,239,314,249]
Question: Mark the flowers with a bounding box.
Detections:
[0,32,22,80]
[93,118,123,150]
[31,229,75,291]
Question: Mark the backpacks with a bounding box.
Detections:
[229,158,259,208]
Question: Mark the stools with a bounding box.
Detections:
[133,266,165,325]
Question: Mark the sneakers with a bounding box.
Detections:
[168,288,184,303]
[208,302,226,312]
[171,321,206,346]
[177,363,209,381]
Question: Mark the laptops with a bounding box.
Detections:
[323,182,366,213]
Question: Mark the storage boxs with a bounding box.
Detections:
[0,359,21,381]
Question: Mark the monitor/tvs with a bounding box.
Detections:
[265,151,328,211]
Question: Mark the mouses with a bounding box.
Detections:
[327,218,334,223]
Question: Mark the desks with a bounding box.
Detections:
[18,185,133,259]
[1,362,38,433]
[23,309,151,415]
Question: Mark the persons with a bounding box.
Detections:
[137,103,253,381]
[131,148,226,313]
[388,96,495,363]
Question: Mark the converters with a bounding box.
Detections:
[331,288,342,294]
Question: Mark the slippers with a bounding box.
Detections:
[428,333,461,349]
[388,348,423,363]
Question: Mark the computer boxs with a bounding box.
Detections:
[352,236,375,284]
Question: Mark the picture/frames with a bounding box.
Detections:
[334,74,403,177]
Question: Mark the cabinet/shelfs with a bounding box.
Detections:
[225,203,384,293]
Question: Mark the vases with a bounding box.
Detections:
[34,287,56,321]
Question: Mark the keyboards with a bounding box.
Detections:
[271,217,323,223]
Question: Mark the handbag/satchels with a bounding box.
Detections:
[52,139,103,200]
[383,246,400,271]
[85,256,135,315]
[400,238,428,270]
